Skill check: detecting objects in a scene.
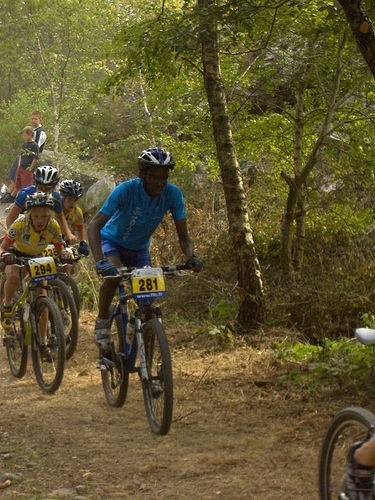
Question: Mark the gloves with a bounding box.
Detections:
[1,251,17,265]
[78,241,91,256]
[61,248,74,261]
[95,258,117,277]
[186,255,204,273]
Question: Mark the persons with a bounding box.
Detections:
[346,431,375,500]
[0,111,89,363]
[87,147,203,396]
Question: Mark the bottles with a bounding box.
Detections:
[126,318,137,345]
[23,298,30,322]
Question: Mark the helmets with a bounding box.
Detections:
[138,147,176,174]
[33,165,60,186]
[59,179,84,198]
[24,191,54,209]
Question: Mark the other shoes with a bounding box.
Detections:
[344,440,375,500]
[95,317,112,351]
[0,192,17,204]
[0,303,14,330]
[41,345,55,360]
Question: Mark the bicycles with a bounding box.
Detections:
[318,327,375,500]
[0,245,92,393]
[99,263,197,435]
